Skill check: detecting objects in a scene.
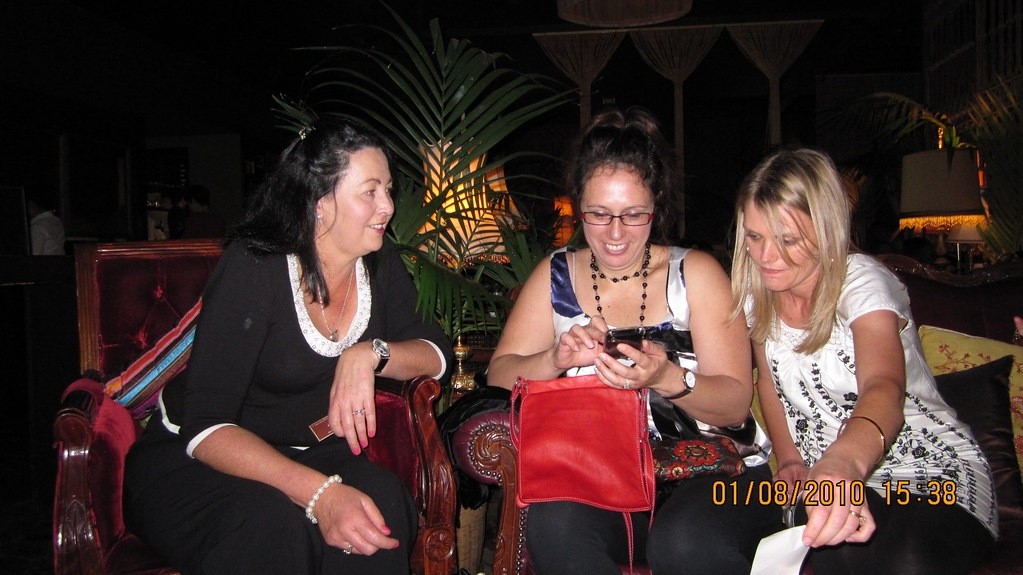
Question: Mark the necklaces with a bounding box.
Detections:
[589,243,652,329]
[318,265,355,342]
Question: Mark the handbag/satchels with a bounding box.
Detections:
[510,376,656,575]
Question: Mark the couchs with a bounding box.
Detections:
[458,255,1023,575]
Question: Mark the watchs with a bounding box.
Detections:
[370,337,391,374]
[662,367,696,400]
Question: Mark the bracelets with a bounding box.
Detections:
[837,417,885,464]
[305,474,343,524]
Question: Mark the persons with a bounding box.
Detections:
[674,194,733,249]
[723,149,998,575]
[123,115,458,575]
[167,185,225,238]
[859,214,938,266]
[486,106,781,575]
[25,197,66,256]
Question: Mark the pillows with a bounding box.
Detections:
[919,323,1023,517]
[103,298,201,431]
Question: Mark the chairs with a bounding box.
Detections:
[50,242,454,575]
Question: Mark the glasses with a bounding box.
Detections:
[576,198,657,226]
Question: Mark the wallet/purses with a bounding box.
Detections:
[651,434,745,479]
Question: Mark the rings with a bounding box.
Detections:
[352,410,364,415]
[343,545,352,555]
[850,510,866,523]
[624,379,629,390]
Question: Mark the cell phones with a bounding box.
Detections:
[602,328,643,360]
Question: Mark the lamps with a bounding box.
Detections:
[416,140,526,398]
[898,146,989,278]
